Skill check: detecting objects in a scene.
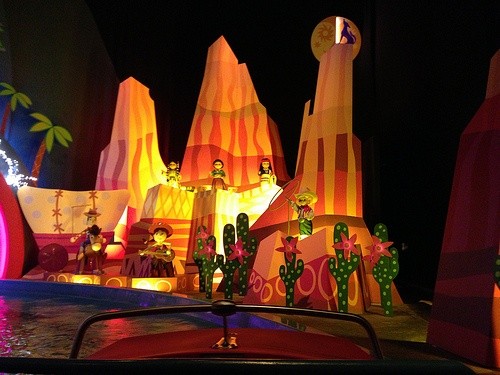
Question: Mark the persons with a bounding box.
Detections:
[258,158,277,186]
[139,222,175,262]
[210,159,226,190]
[162,158,181,187]
[289,191,318,239]
